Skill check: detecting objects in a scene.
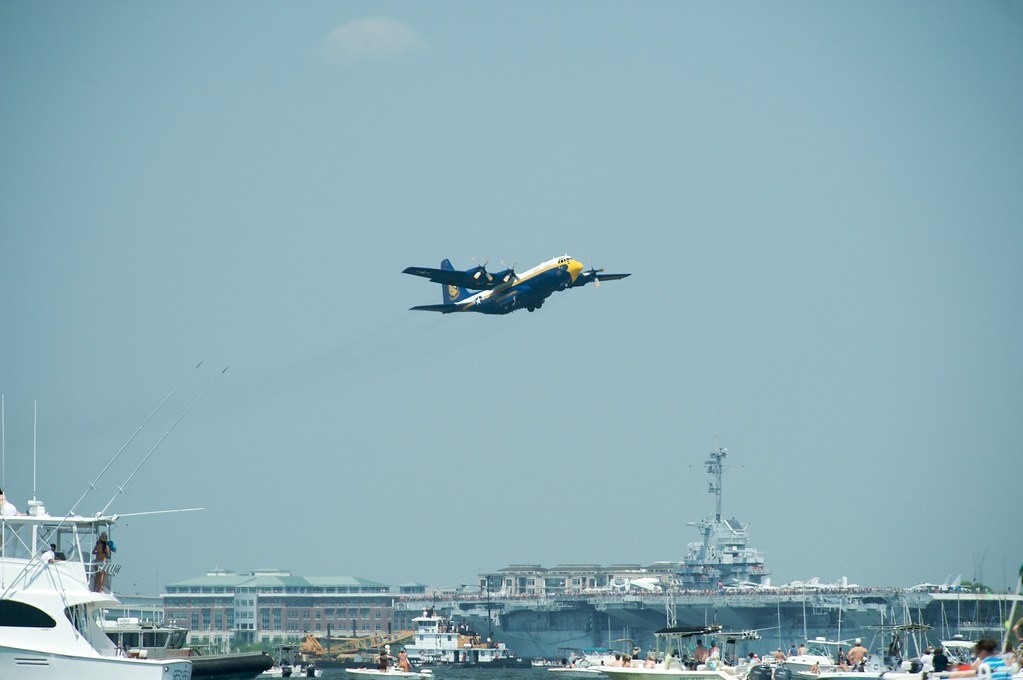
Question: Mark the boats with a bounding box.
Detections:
[92,571,273,680]
[262,641,323,678]
[0,361,232,680]
[546,584,1023,680]
[345,644,435,680]
[389,591,532,669]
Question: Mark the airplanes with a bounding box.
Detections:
[401,253,632,314]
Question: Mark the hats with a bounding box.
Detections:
[856,638,861,645]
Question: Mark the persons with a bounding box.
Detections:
[570,660,576,668]
[888,635,902,665]
[672,650,697,670]
[438,619,480,645]
[612,655,622,667]
[0,488,29,516]
[397,648,408,672]
[897,646,948,680]
[971,617,1023,680]
[426,604,434,618]
[622,653,638,668]
[49,544,62,563]
[798,643,807,655]
[746,652,760,664]
[508,654,517,659]
[376,650,393,673]
[632,647,641,659]
[811,661,820,674]
[92,531,111,594]
[694,639,720,670]
[790,644,797,656]
[952,651,966,672]
[503,649,509,656]
[838,638,868,672]
[488,641,498,648]
[643,655,655,669]
[775,648,785,661]
